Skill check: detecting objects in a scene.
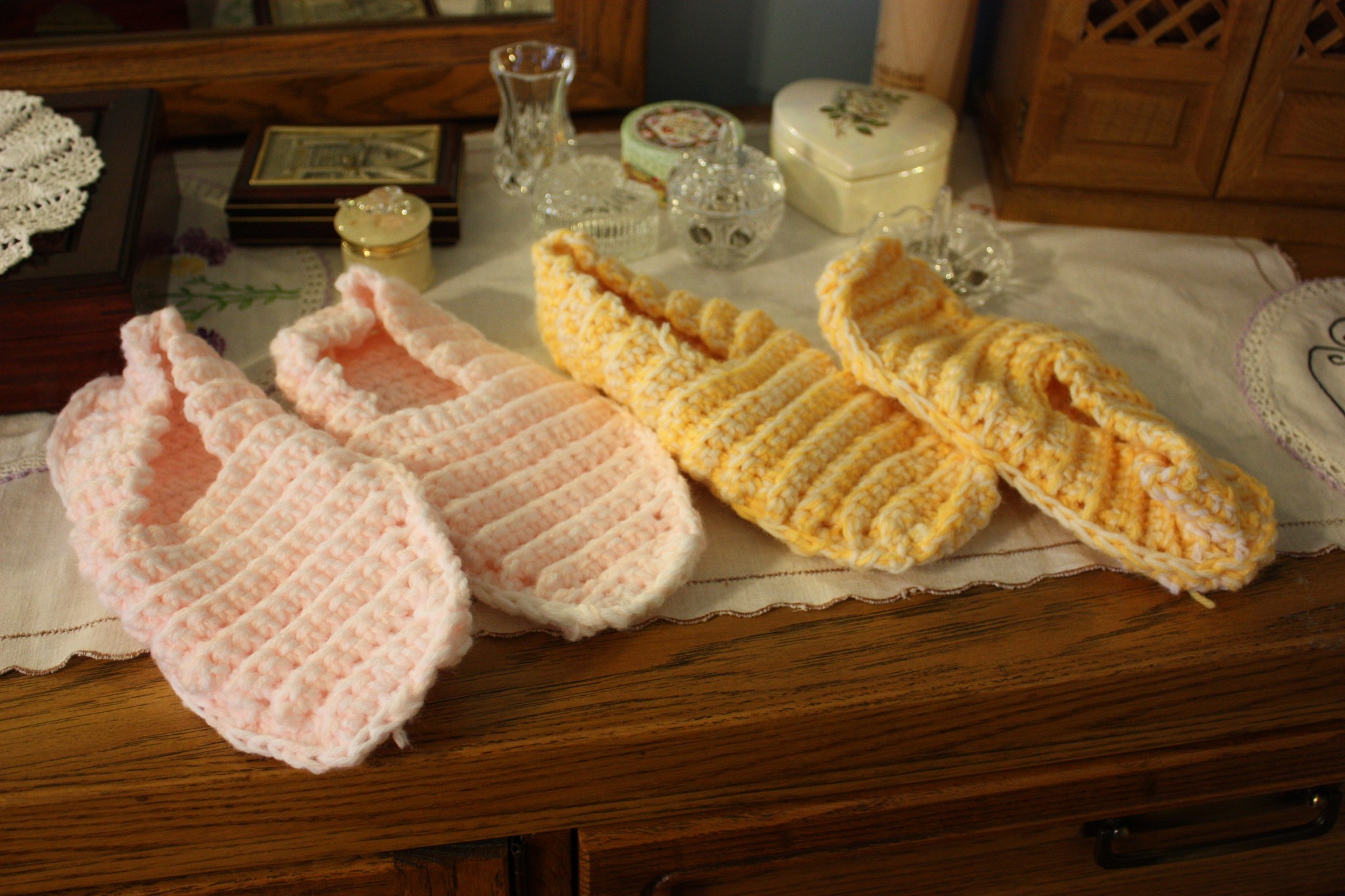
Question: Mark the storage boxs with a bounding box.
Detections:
[771,79,957,234]
[0,88,167,291]
[224,124,464,247]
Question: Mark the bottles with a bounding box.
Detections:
[486,41,577,196]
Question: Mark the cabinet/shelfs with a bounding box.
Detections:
[0,121,1345,896]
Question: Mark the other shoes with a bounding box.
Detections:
[45,304,475,774]
[531,226,1003,574]
[810,235,1279,610]
[269,264,708,637]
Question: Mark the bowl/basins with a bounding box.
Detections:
[860,184,1015,313]
